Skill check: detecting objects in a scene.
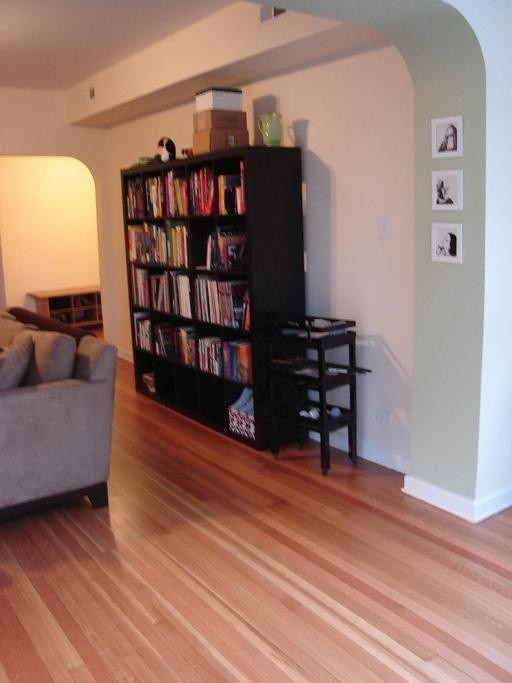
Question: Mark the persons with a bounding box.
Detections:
[436,232,457,256]
[439,125,457,151]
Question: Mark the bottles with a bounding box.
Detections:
[182,148,192,157]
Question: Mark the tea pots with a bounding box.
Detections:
[257,111,282,147]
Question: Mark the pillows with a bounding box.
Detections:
[0,333,35,392]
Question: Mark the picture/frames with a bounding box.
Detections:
[430,113,464,160]
[429,219,465,266]
[431,167,465,213]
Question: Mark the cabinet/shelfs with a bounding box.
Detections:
[268,311,360,480]
[117,140,311,454]
[25,284,102,332]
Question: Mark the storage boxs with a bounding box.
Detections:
[225,405,255,441]
[187,87,251,155]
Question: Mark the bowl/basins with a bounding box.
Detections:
[141,371,155,394]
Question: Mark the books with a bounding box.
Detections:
[124,160,253,386]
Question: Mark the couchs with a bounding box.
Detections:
[1,304,120,513]
